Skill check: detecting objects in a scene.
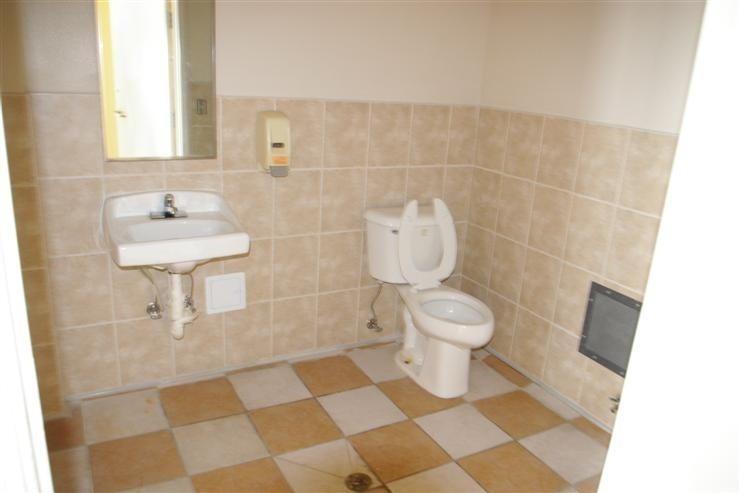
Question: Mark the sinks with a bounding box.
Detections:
[104,212,250,268]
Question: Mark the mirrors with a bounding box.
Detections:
[95,0,217,162]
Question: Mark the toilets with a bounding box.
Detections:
[363,197,494,398]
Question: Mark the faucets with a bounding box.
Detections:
[164,194,178,217]
